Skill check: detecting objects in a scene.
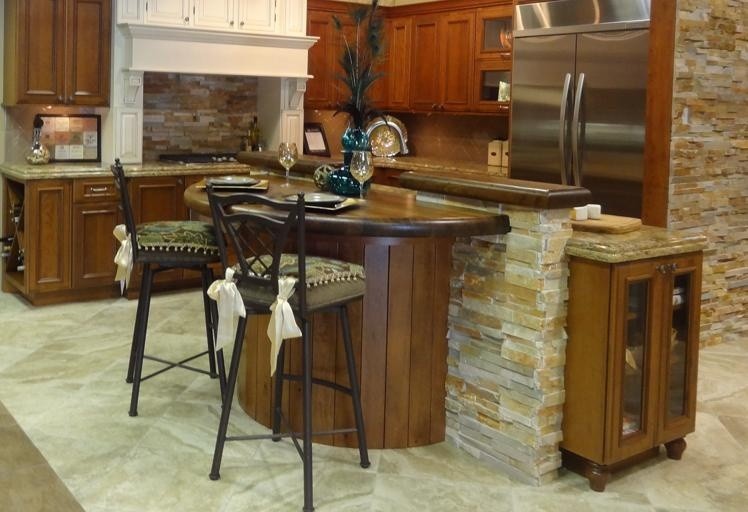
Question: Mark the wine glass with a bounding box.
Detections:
[277,142,297,188]
[349,150,373,204]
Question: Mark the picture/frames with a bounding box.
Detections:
[300,122,332,157]
[34,113,102,162]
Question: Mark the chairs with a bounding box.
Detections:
[108,159,229,419]
[206,179,370,508]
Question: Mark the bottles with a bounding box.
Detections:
[0,202,26,272]
[244,117,265,153]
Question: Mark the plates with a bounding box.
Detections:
[207,174,260,186]
[288,192,344,204]
[366,115,408,158]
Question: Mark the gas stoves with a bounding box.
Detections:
[158,153,239,163]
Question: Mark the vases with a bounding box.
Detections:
[25,118,51,166]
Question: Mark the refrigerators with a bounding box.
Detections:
[508,1,651,219]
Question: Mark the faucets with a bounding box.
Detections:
[365,120,408,155]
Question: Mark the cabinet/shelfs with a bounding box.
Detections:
[112,0,190,38]
[307,1,515,117]
[193,0,285,35]
[559,251,705,491]
[1,1,111,109]
[3,174,248,305]
